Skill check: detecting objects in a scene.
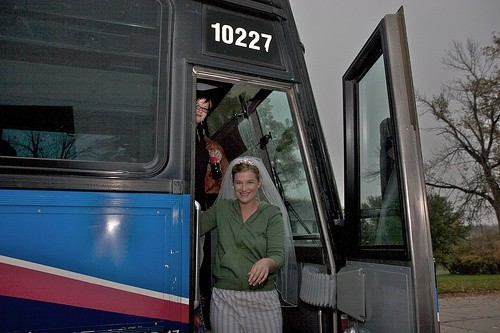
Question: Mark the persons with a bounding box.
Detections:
[204,134,229,208]
[199,156,285,333]
[194,94,222,299]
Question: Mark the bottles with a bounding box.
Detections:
[210,146,221,179]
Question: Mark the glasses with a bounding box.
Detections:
[195,104,209,113]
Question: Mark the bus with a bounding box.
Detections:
[0,0,442,333]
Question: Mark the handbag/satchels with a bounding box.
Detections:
[204,162,220,193]
